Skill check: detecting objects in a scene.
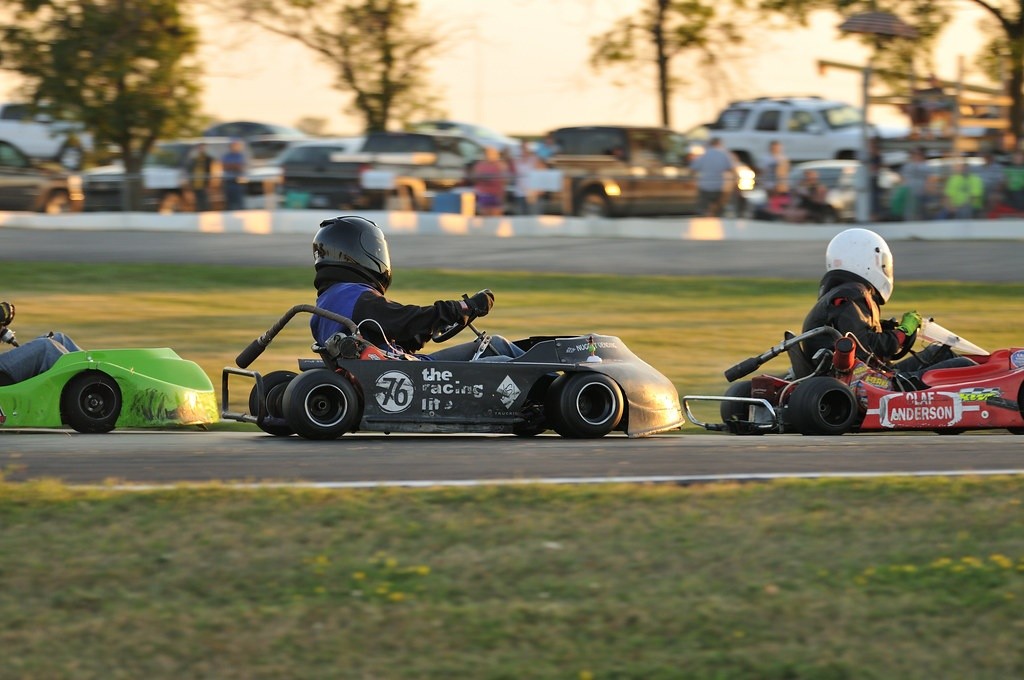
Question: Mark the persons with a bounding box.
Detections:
[802,170,827,209]
[58,133,83,171]
[474,147,510,217]
[974,151,1024,210]
[310,215,526,361]
[223,137,250,211]
[689,137,739,216]
[902,148,932,220]
[0,301,83,387]
[932,158,983,219]
[502,133,563,215]
[761,140,790,192]
[187,143,216,211]
[868,135,882,213]
[802,228,978,393]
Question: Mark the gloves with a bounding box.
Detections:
[462,289,495,317]
[895,310,922,336]
[0,302,15,325]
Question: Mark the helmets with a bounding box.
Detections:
[818,228,894,305]
[313,216,391,295]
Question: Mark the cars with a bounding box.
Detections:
[231,122,1019,216]
[0,97,98,171]
[142,117,317,164]
[0,138,84,216]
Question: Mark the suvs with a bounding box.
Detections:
[82,135,249,215]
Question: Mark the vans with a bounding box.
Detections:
[703,95,880,170]
